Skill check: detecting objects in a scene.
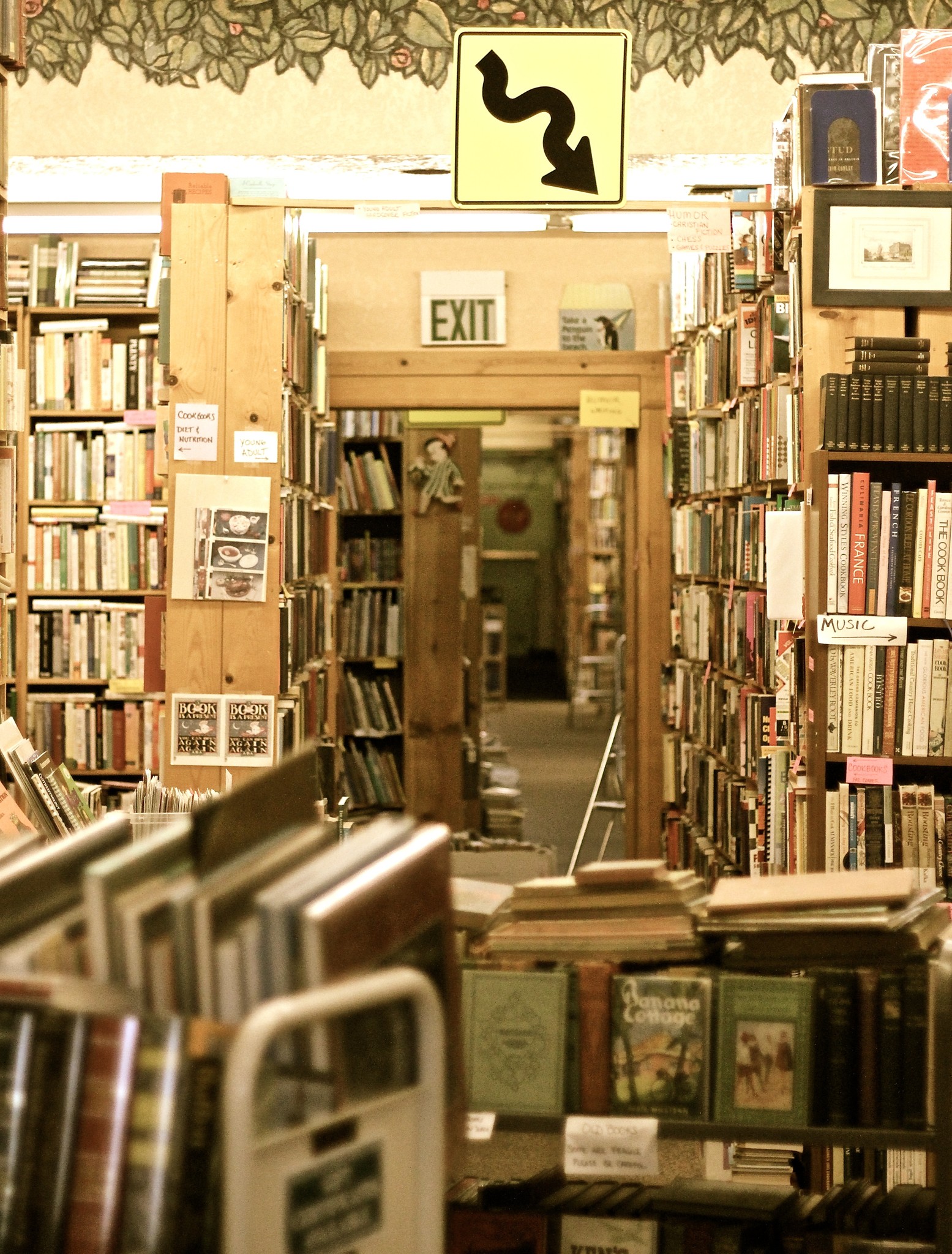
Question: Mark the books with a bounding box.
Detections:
[0,26,950,1254]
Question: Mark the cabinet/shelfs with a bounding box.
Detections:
[0,170,952,1252]
